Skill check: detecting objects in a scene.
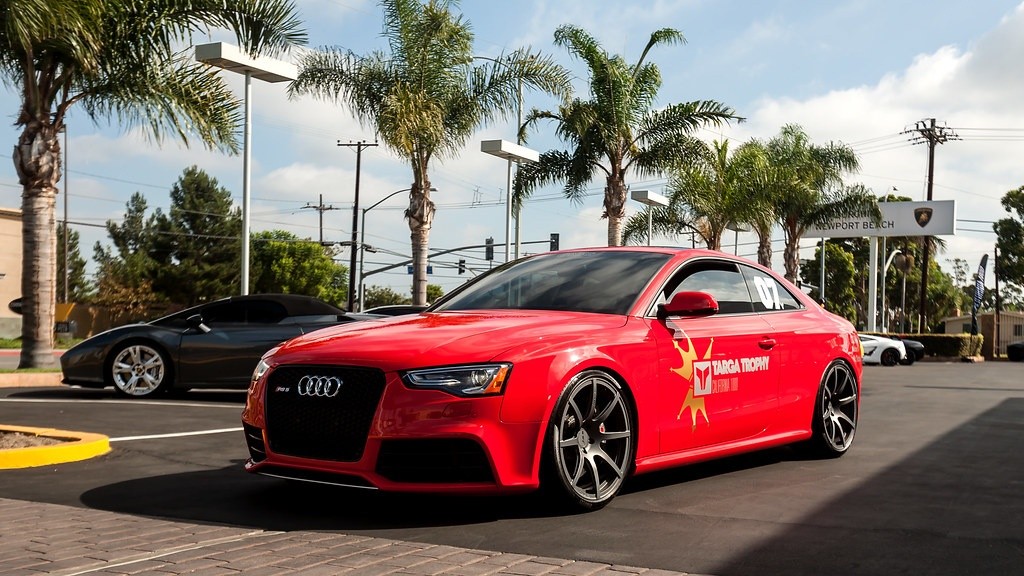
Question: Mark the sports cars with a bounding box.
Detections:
[57,293,432,400]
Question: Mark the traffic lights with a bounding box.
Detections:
[458,259,466,274]
[818,297,826,309]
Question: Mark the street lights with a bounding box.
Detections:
[58,123,71,305]
[630,190,670,246]
[879,186,901,333]
[358,186,439,313]
[727,221,751,256]
[480,139,540,262]
[194,41,300,295]
[452,54,523,259]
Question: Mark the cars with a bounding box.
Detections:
[870,333,926,366]
[857,333,908,367]
[238,244,864,513]
